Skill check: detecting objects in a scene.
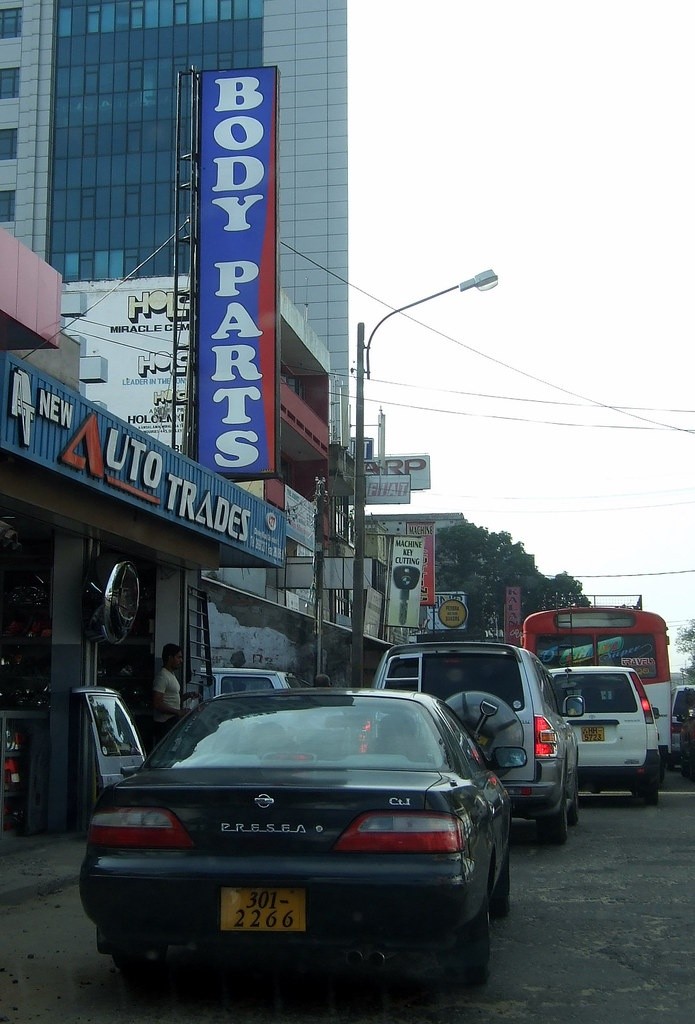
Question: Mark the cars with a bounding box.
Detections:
[675,686,694,780]
[80,685,531,1001]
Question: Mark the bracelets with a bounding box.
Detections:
[189,692,192,697]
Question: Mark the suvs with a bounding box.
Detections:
[371,641,587,844]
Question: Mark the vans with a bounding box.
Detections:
[671,685,695,753]
[536,665,666,805]
[201,667,311,698]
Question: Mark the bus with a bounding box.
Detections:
[521,594,674,775]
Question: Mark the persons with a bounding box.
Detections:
[151,643,202,745]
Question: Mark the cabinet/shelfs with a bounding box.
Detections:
[0,565,53,837]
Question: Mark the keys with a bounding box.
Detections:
[394,565,420,626]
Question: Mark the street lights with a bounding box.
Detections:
[350,271,505,686]
